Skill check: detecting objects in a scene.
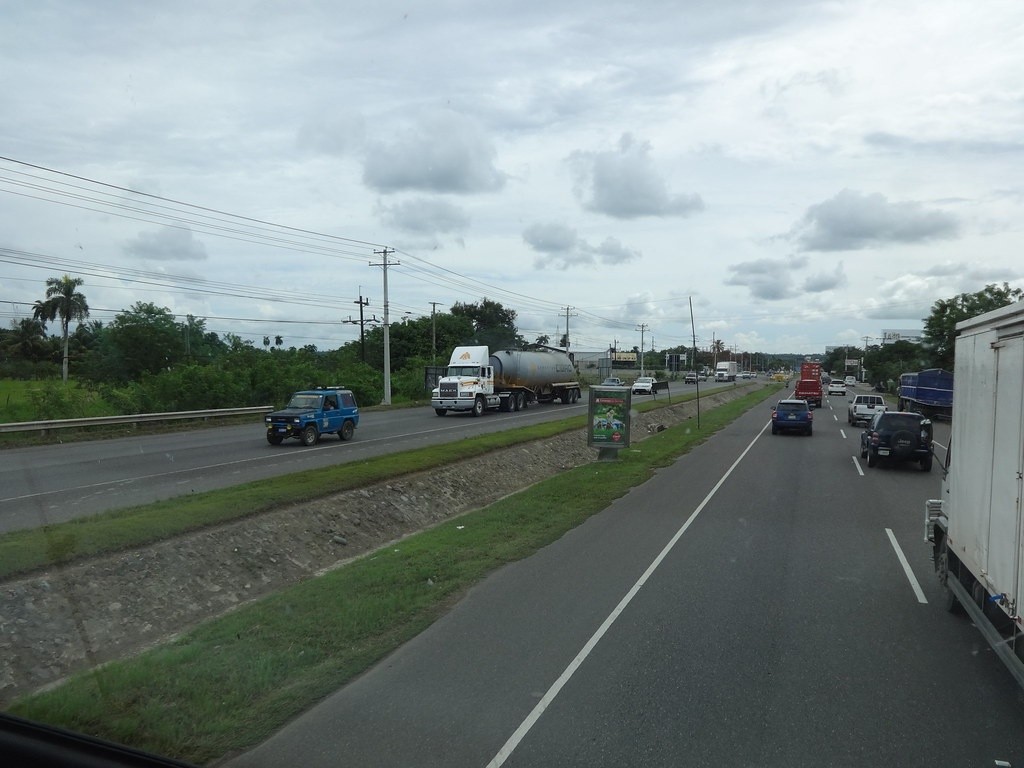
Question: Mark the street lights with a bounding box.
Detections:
[429,302,444,367]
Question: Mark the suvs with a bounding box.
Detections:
[264,386,359,446]
[859,408,934,471]
[770,399,815,437]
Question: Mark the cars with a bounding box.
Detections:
[742,371,751,379]
[828,379,847,396]
[697,373,707,381]
[750,372,757,378]
[601,378,624,386]
[845,376,856,386]
[819,368,831,385]
[761,371,765,375]
[685,373,696,384]
[631,377,657,394]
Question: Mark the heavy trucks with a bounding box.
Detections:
[430,345,582,416]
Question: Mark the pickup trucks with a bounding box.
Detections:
[847,395,888,428]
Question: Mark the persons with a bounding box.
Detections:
[312,399,334,410]
[897,379,901,385]
[875,379,885,393]
[477,369,483,377]
[597,408,614,430]
[611,431,624,442]
[488,373,491,377]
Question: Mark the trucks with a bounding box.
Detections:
[898,368,954,422]
[801,362,821,381]
[922,298,1024,688]
[714,361,738,382]
[794,379,823,409]
